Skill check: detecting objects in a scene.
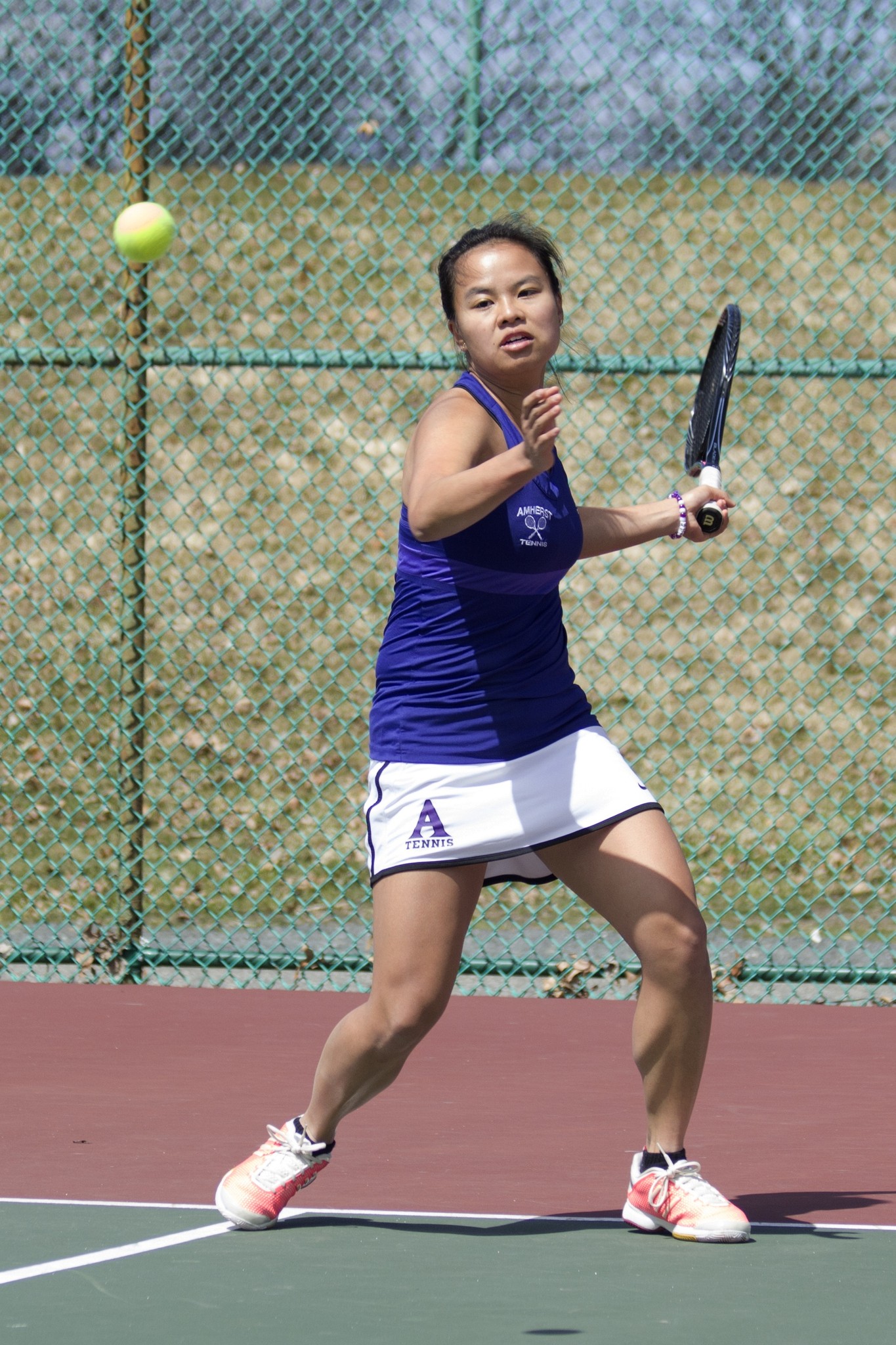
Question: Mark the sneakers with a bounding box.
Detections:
[621,1143,751,1243]
[215,1113,332,1230]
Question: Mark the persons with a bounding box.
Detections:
[213,222,753,1242]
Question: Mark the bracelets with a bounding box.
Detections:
[668,492,686,540]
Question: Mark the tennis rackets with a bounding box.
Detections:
[684,302,742,535]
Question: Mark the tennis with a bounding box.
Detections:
[110,201,182,266]
